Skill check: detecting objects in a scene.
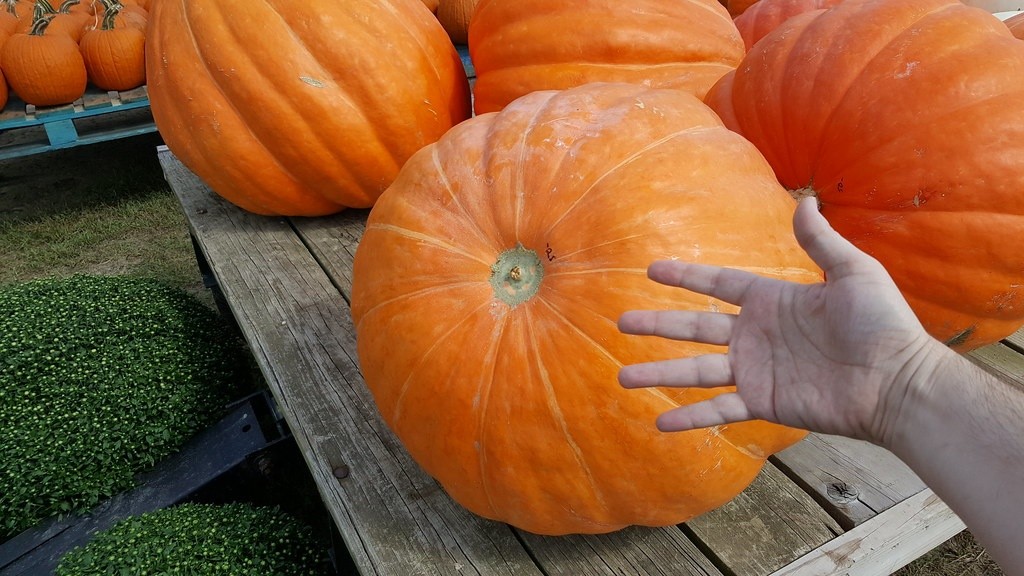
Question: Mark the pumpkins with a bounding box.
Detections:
[0,0,1024,353]
[352,80,826,535]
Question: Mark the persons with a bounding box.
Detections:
[612,193,1022,574]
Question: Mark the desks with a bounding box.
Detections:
[156,141,1024,576]
